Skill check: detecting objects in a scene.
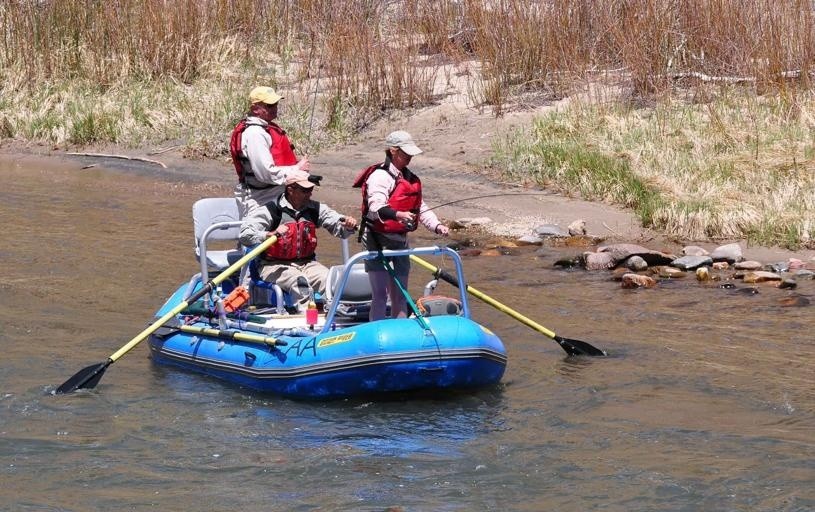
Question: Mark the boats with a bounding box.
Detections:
[149,276,506,398]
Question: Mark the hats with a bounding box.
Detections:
[284,170,316,188]
[248,86,284,105]
[383,131,423,155]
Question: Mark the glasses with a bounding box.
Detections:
[260,101,277,109]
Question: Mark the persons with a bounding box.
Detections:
[353,130,450,322]
[239,170,358,316]
[230,86,311,291]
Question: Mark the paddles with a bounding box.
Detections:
[339,218,606,357]
[49,231,282,395]
